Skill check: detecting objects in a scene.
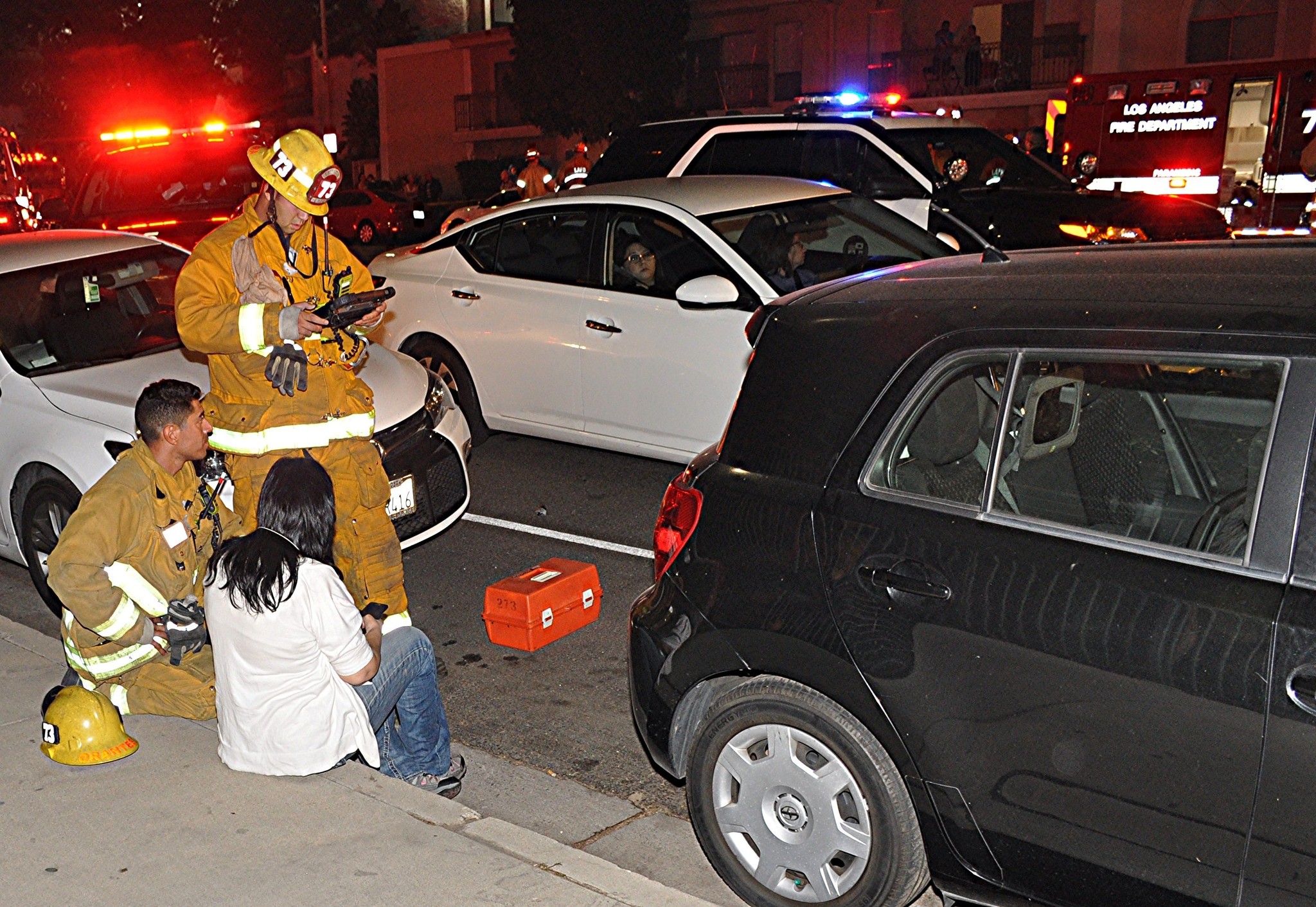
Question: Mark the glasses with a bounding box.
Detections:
[622,251,655,263]
[790,240,804,248]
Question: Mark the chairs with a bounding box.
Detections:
[35,268,126,363]
[890,362,1316,575]
[480,213,795,295]
[922,60,1012,97]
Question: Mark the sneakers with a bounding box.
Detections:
[441,754,466,779]
[404,772,462,800]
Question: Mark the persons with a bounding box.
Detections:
[961,24,982,95]
[980,127,1062,190]
[500,140,592,200]
[202,455,467,800]
[46,378,247,722]
[749,214,819,293]
[358,172,443,204]
[174,128,412,638]
[618,236,676,299]
[933,20,959,73]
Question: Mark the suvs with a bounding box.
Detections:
[585,87,1238,255]
[625,254,1316,907]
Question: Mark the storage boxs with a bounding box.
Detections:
[482,557,603,653]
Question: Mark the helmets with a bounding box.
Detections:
[573,143,586,152]
[40,685,140,765]
[525,148,540,158]
[247,128,343,216]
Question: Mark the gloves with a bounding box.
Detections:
[264,339,308,396]
[163,599,207,666]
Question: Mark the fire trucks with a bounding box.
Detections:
[0,116,274,307]
[1043,52,1316,240]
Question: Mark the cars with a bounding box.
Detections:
[366,171,964,468]
[440,187,522,235]
[312,188,415,247]
[0,227,475,623]
